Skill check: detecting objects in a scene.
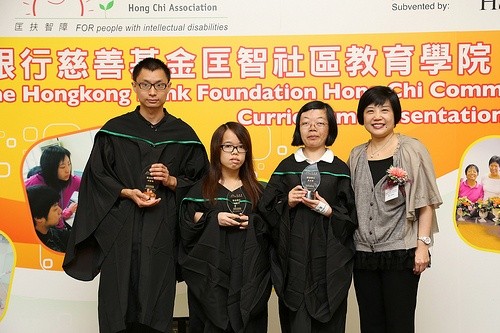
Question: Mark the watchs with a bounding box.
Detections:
[417,236,432,246]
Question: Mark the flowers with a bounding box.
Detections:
[382,165,413,197]
[456,196,475,216]
[475,197,500,213]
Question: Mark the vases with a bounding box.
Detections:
[457,208,465,221]
[478,209,500,222]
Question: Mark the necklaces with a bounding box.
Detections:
[369,131,396,159]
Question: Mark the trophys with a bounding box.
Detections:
[139,165,157,201]
[225,189,246,223]
[299,163,321,202]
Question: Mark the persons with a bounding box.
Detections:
[178,121,270,333]
[250,99,359,333]
[345,86,443,333]
[60,56,212,333]
[459,155,500,211]
[25,145,82,252]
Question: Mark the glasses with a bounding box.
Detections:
[135,80,170,90]
[300,120,328,127]
[219,144,248,152]
